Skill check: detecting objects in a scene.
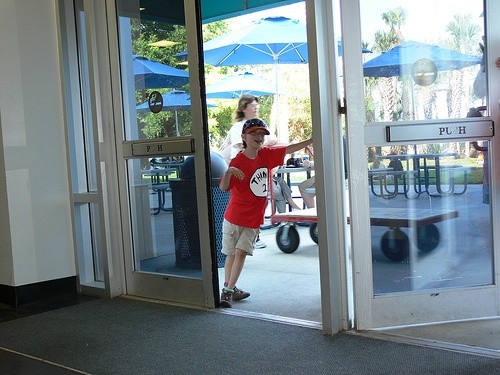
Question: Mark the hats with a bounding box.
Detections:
[242,118,270,135]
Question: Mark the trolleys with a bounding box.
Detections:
[270,162,458,262]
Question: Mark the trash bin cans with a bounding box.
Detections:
[169,153,230,268]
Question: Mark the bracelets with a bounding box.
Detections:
[309,161,312,167]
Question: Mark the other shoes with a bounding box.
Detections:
[233,286,250,301]
[220,287,234,308]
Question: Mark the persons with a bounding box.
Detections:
[369,155,386,180]
[298,134,349,208]
[387,152,404,179]
[218,118,314,308]
[227,94,277,158]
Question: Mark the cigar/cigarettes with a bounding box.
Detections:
[300,163,303,165]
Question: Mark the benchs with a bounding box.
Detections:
[149,182,173,215]
[368,165,468,199]
[305,178,349,194]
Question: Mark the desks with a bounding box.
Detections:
[379,153,456,198]
[140,168,178,215]
[273,165,312,229]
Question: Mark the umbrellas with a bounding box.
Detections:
[126,54,190,89]
[136,88,219,180]
[195,70,286,100]
[358,41,484,194]
[172,15,372,214]
